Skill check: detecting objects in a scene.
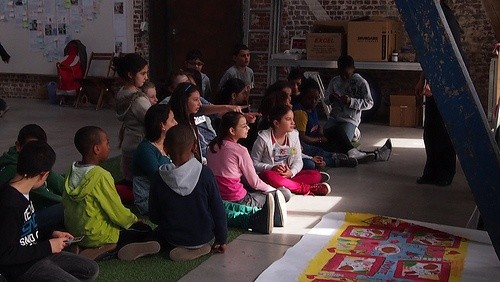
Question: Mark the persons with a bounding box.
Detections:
[0,45,392,282]
[414,72,456,187]
[0,43,11,117]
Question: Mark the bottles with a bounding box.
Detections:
[391,52,398,62]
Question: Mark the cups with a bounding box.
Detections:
[115,41,122,53]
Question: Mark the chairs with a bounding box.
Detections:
[73,52,114,109]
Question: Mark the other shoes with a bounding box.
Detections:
[118,241,160,260]
[79,243,117,262]
[417,175,452,186]
[334,154,358,168]
[0,105,10,117]
[348,149,367,159]
[169,244,211,262]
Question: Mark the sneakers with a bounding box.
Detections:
[309,183,332,197]
[320,172,330,182]
[374,138,392,161]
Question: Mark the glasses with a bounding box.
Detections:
[187,61,203,67]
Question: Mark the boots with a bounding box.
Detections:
[251,193,274,234]
[273,189,287,227]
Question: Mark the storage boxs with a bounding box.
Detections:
[389,95,417,128]
[305,32,343,62]
[345,17,401,62]
